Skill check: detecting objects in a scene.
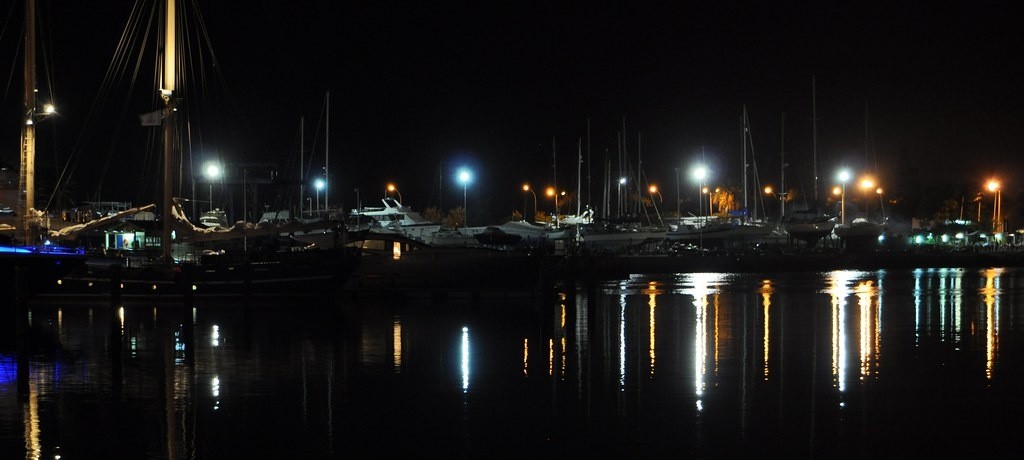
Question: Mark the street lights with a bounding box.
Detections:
[313,178,325,207]
[455,170,468,225]
[837,168,851,224]
[987,181,1002,229]
[200,160,221,212]
[688,164,707,215]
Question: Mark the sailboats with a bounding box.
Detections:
[0,0,1024,304]
